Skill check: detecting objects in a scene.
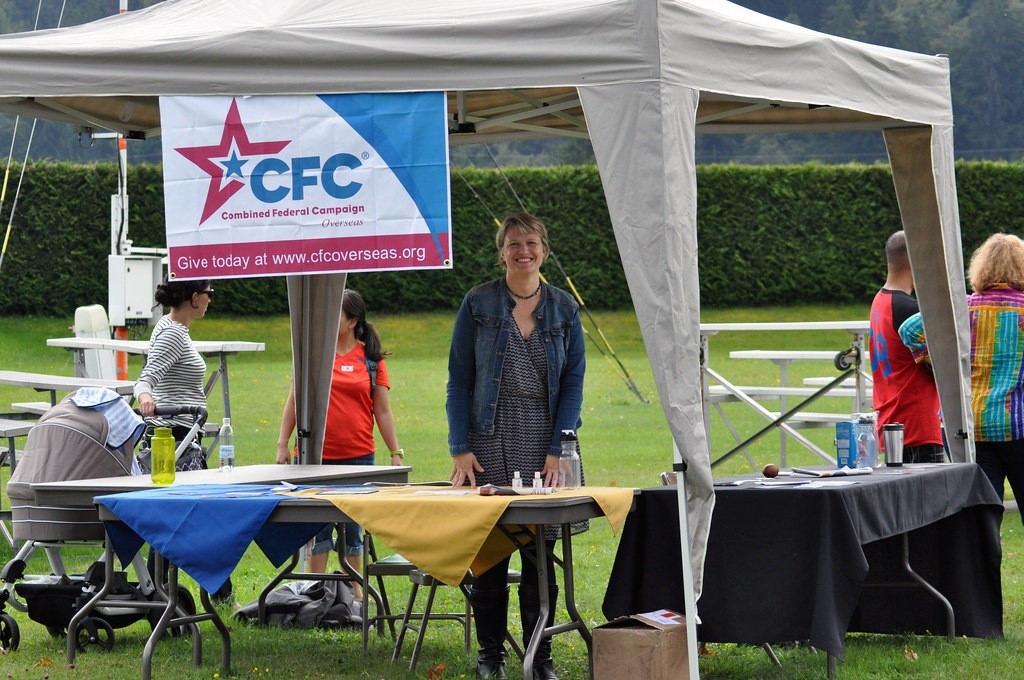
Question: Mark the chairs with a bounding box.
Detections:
[363,480,525,671]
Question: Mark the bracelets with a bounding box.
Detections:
[279,438,290,442]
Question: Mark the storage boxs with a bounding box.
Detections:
[836,421,876,468]
[592,609,690,680]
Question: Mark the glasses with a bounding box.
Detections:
[197,288,215,299]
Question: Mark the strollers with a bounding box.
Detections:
[0,387,209,656]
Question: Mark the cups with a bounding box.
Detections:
[883,422,904,467]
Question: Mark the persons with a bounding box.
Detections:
[868,229,948,464]
[133,269,244,618]
[444,211,586,680]
[897,234,1024,524]
[276,288,404,602]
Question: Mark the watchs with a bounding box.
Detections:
[389,448,405,457]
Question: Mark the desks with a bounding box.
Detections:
[30,464,413,669]
[92,484,641,680]
[602,462,1005,680]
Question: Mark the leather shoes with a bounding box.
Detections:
[475,662,509,680]
[532,656,560,680]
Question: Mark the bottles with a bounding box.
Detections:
[512,471,523,489]
[151,427,176,484]
[559,435,580,489]
[533,472,542,488]
[857,420,875,467]
[218,418,234,472]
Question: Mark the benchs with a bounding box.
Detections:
[0,336,265,521]
[698,320,874,474]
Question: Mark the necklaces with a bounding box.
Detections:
[506,282,543,299]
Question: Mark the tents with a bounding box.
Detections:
[0,0,977,680]
